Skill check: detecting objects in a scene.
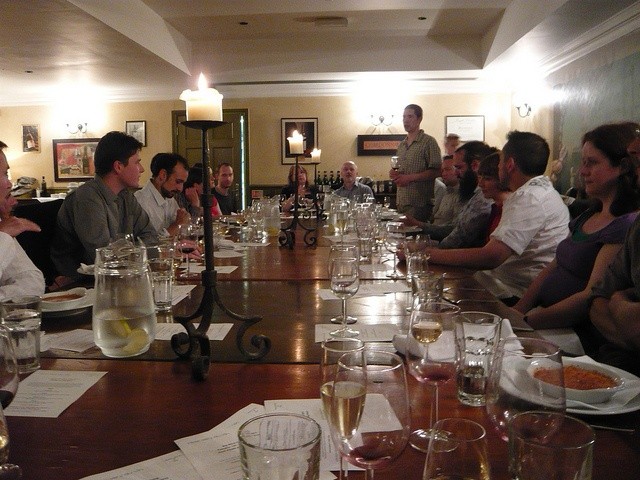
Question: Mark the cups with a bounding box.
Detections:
[154,275,173,312]
[178,224,192,241]
[158,244,173,276]
[1,294,40,320]
[0,327,20,409]
[423,418,490,480]
[238,414,322,480]
[411,274,443,312]
[386,222,404,239]
[506,410,596,480]
[3,309,41,374]
[455,311,502,408]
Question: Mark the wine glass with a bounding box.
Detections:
[330,351,411,480]
[404,235,434,277]
[0,403,23,480]
[383,231,406,277]
[354,211,376,262]
[330,198,382,220]
[407,302,466,454]
[384,197,390,211]
[74,148,81,165]
[410,303,444,374]
[290,194,300,211]
[335,210,350,243]
[177,231,198,278]
[486,337,565,480]
[320,338,367,480]
[390,156,401,181]
[218,215,229,240]
[406,256,429,312]
[327,245,358,324]
[330,258,360,338]
[252,205,264,239]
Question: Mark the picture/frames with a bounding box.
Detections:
[51,138,102,183]
[358,134,407,156]
[280,117,319,165]
[125,120,147,148]
[19,122,41,154]
[445,115,486,144]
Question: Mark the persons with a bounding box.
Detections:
[388,104,442,223]
[134,153,192,240]
[47,132,157,283]
[397,130,571,307]
[444,134,459,156]
[580,131,640,377]
[279,165,316,212]
[428,155,464,226]
[0,191,18,219]
[26,129,35,148]
[0,141,45,303]
[469,152,513,249]
[211,161,237,215]
[400,141,500,248]
[176,166,203,224]
[334,161,374,203]
[432,177,447,217]
[195,162,223,216]
[514,123,640,361]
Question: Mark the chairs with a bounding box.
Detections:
[8,198,62,287]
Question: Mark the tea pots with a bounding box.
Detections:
[93,246,157,358]
[256,195,282,242]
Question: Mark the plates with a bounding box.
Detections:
[527,358,624,403]
[499,356,640,415]
[40,287,87,310]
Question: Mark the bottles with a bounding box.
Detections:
[322,171,329,193]
[82,146,89,174]
[379,175,385,193]
[372,175,377,194]
[300,125,306,154]
[388,181,393,193]
[316,171,322,193]
[335,171,343,190]
[41,176,46,190]
[329,170,335,193]
[26,128,36,149]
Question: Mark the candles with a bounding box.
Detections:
[178,71,226,122]
[309,148,322,163]
[287,129,304,155]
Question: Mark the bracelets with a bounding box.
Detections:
[523,316,528,323]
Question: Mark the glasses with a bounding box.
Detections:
[454,161,470,170]
